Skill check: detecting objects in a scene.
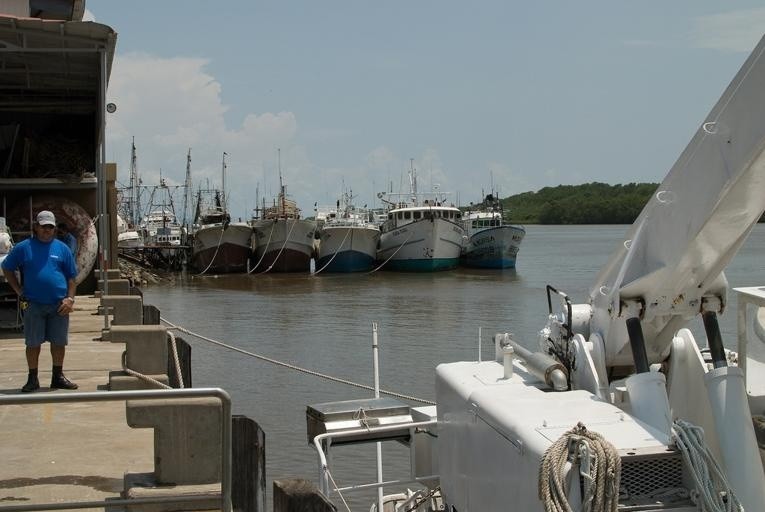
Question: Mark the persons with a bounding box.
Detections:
[0,209,80,393]
[55,223,78,261]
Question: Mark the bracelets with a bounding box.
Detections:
[68,296,75,304]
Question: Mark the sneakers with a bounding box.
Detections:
[22,378,40,392]
[51,374,78,389]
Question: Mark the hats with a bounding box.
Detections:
[36,211,56,227]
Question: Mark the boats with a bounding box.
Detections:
[246,149,317,273]
[461,168,526,271]
[295,27,765,512]
[114,135,189,275]
[186,151,252,275]
[312,180,381,274]
[378,158,466,274]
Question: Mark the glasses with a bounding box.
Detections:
[38,223,55,229]
